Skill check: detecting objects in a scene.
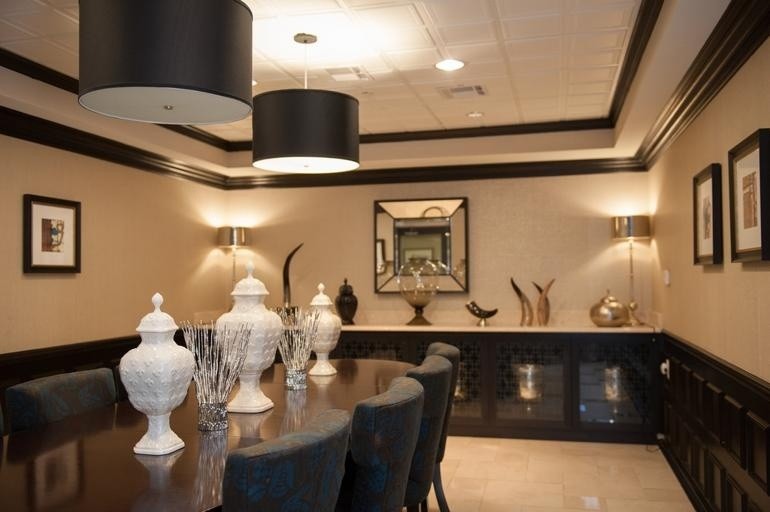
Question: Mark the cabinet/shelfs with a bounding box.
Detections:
[570,341,646,424]
[485,339,569,425]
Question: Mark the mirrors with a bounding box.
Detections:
[373,196,469,294]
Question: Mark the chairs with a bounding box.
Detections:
[5,341,458,512]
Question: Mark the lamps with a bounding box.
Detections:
[251,33,360,176]
[611,215,652,323]
[77,0,254,126]
[217,225,247,309]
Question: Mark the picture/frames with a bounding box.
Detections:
[23,194,81,273]
[727,128,770,262]
[692,163,723,265]
